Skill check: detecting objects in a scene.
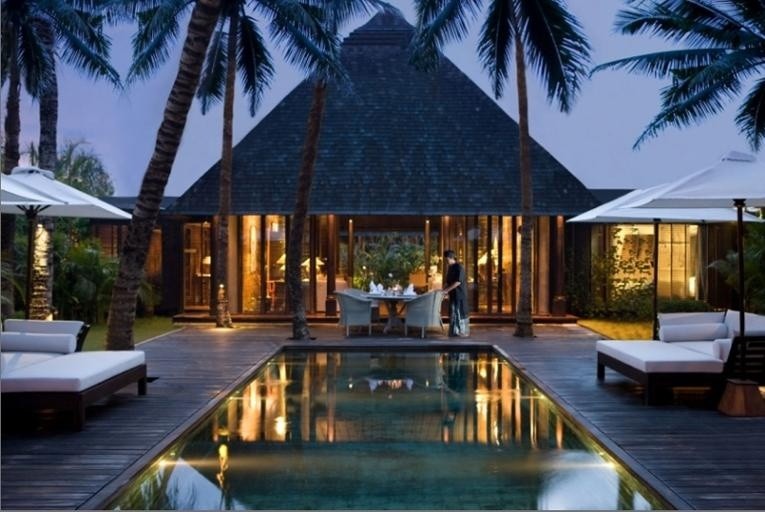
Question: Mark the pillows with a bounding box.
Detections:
[659,321,747,359]
[1,332,76,353]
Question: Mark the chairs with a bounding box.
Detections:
[0,315,90,420]
[333,287,447,339]
[653,307,765,408]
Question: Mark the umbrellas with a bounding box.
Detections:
[615,148,764,381]
[0,166,134,317]
[0,171,67,209]
[568,183,765,335]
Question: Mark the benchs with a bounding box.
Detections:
[1,349,146,429]
[596,339,723,407]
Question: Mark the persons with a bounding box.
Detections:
[439,249,472,338]
[441,353,471,429]
